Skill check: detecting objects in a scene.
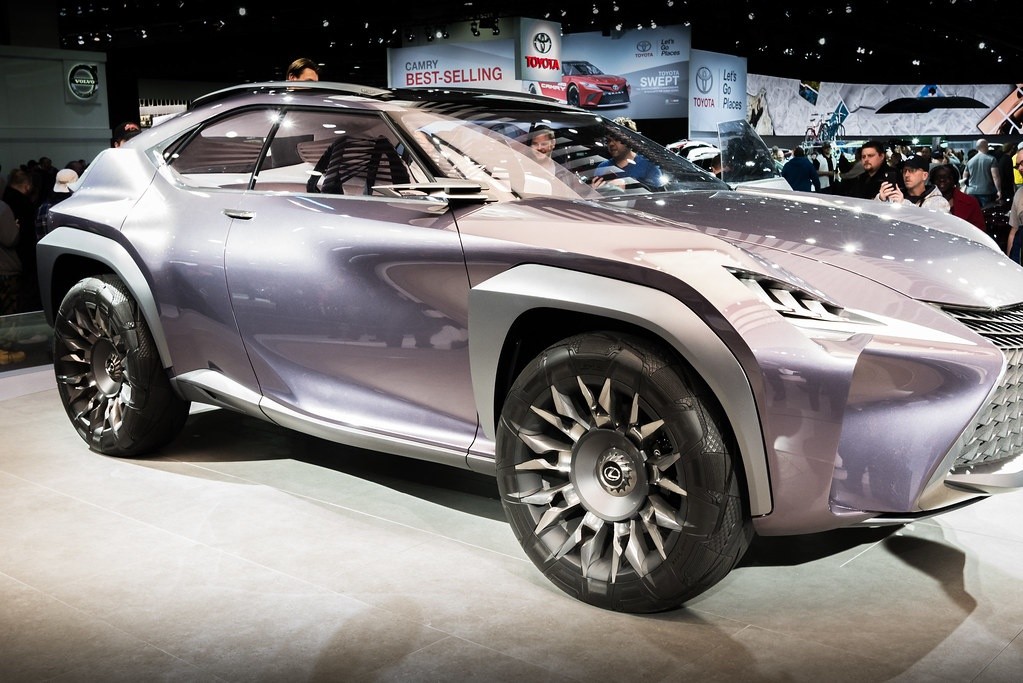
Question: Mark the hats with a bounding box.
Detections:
[53,169,78,192]
[113,122,141,139]
[900,154,929,173]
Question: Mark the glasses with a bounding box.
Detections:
[901,167,922,174]
[1013,160,1023,170]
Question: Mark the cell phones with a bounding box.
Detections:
[595,169,618,183]
[884,172,896,190]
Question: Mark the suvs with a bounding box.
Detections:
[34,81,1023,615]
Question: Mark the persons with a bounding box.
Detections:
[1007,148,1023,257]
[287,58,318,91]
[519,125,579,198]
[875,156,951,214]
[930,164,986,234]
[773,139,1023,200]
[846,142,904,202]
[589,116,666,192]
[0,156,91,366]
[963,139,1002,207]
[111,116,141,149]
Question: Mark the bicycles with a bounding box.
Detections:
[805,112,848,146]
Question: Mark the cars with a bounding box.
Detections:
[529,61,631,108]
